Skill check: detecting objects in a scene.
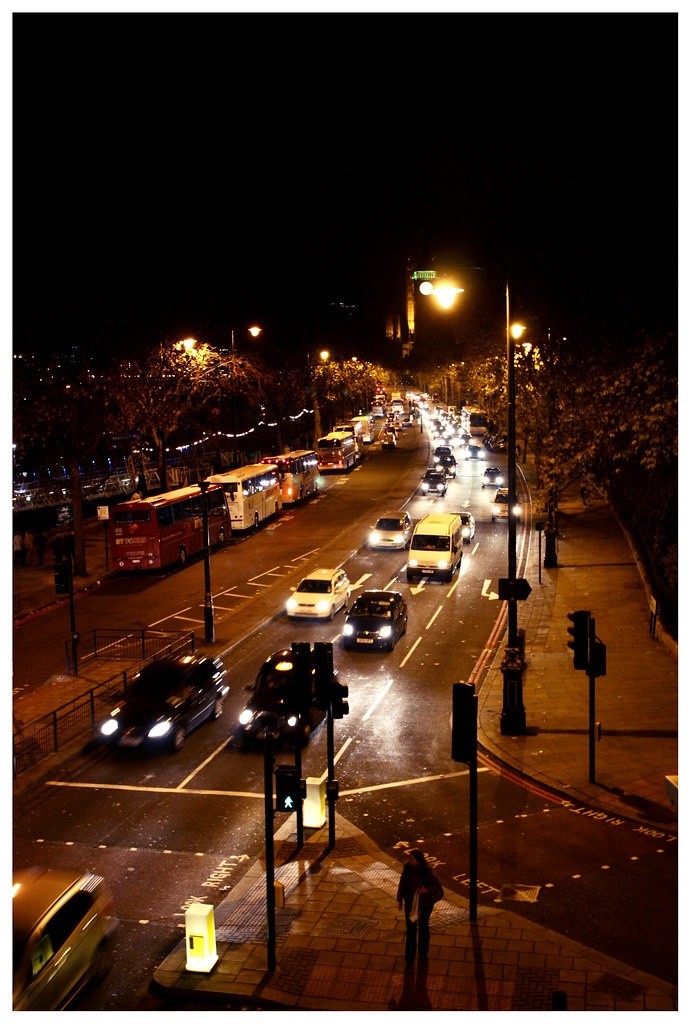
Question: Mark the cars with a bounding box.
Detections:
[12,864,119,1011]
[410,394,504,488]
[449,512,475,544]
[369,512,413,549]
[342,589,407,652]
[240,648,340,749]
[381,411,412,448]
[100,651,228,752]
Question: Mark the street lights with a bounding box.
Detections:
[231,323,262,468]
[431,266,527,736]
[511,317,558,568]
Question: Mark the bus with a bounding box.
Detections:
[460,405,488,434]
[109,450,318,572]
[317,385,404,472]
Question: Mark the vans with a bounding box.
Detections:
[408,514,462,581]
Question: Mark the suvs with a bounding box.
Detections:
[420,468,448,496]
[286,568,350,621]
[490,487,521,522]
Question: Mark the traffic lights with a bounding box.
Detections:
[567,611,587,669]
[275,766,300,812]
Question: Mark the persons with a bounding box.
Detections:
[396,849,444,966]
